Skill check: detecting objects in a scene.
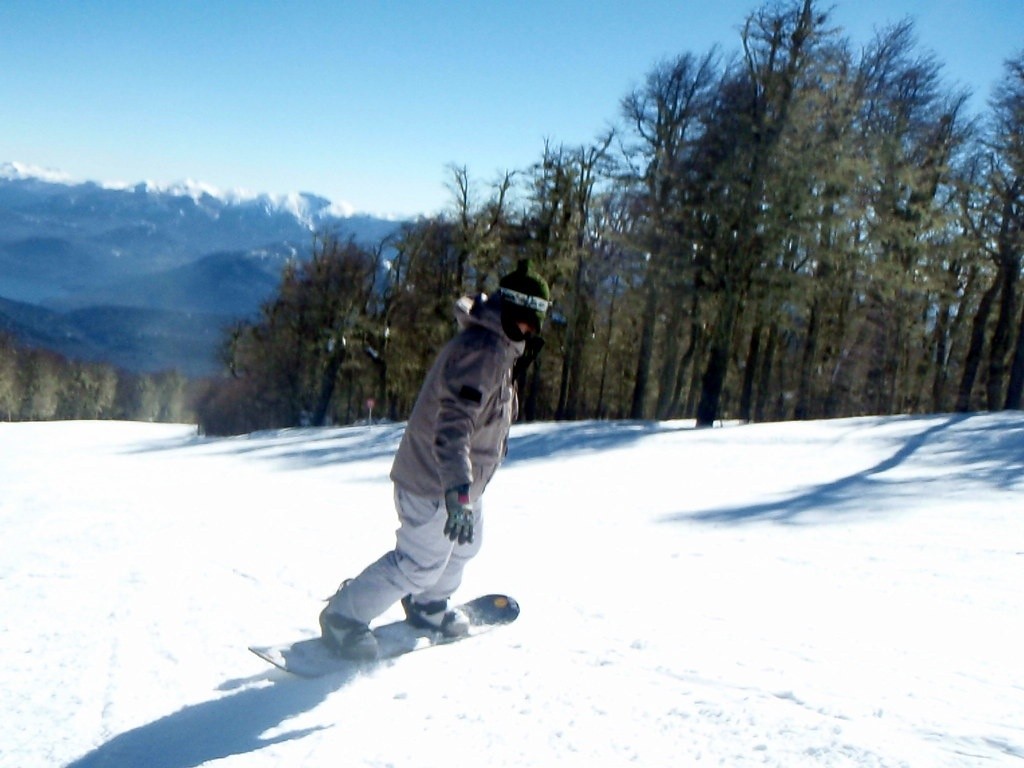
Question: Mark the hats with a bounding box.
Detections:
[497,269,549,331]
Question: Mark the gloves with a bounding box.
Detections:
[444,484,475,545]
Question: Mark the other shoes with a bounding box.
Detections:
[320,609,379,659]
[409,595,468,637]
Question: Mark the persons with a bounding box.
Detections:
[319,274,549,657]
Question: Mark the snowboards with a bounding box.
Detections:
[246,593,520,679]
